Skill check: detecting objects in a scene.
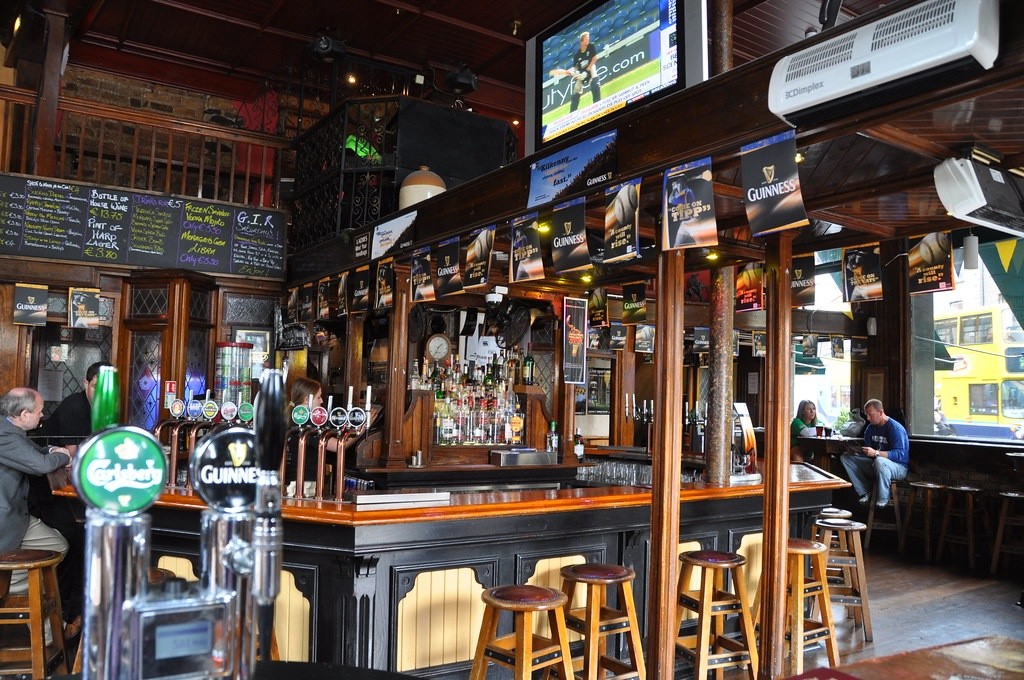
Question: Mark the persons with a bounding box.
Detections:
[284,378,377,483]
[669,177,703,247]
[413,257,432,302]
[0,386,82,654]
[513,227,533,281]
[569,32,601,113]
[840,400,910,510]
[33,362,113,610]
[790,401,841,461]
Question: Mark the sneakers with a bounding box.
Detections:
[876,498,890,508]
[858,491,872,502]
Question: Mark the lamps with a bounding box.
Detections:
[963,226,978,269]
[867,317,877,336]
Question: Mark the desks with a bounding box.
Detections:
[799,436,1024,475]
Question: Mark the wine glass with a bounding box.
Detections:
[737,455,751,475]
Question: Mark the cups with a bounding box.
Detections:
[825,425,832,437]
[815,423,823,437]
[575,457,701,490]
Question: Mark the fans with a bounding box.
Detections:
[487,298,531,349]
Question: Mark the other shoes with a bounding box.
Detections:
[47,615,81,648]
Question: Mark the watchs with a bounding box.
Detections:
[875,450,879,458]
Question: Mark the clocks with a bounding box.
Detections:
[425,333,452,363]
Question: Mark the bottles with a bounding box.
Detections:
[545,420,559,456]
[410,341,536,445]
[573,427,585,464]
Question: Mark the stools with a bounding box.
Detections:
[864,478,1024,576]
[811,507,873,643]
[738,537,841,678]
[542,563,647,680]
[469,584,575,680]
[0,549,69,680]
[676,550,759,680]
